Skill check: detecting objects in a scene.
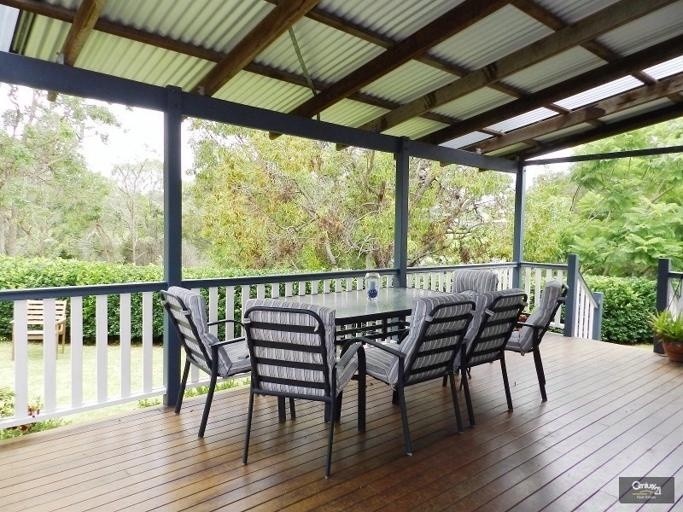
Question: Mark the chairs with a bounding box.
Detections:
[161,270,565,481]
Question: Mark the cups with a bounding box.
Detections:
[364,271,381,303]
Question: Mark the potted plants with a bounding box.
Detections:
[643,306,682,362]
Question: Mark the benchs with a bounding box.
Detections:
[9,300,67,354]
